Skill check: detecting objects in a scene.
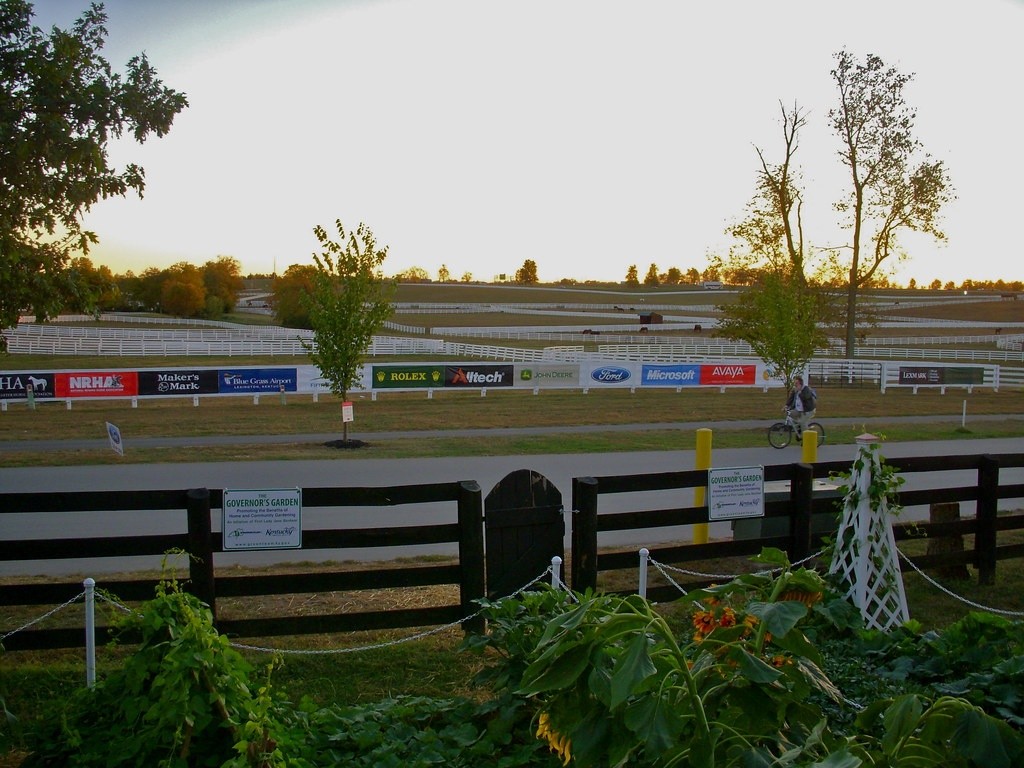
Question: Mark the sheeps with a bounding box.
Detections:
[639,327,648,334]
[583,329,600,336]
[694,324,702,333]
[894,301,899,306]
[614,305,624,313]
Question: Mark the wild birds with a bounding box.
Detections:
[630,308,635,313]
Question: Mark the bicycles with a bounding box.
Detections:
[768,406,825,449]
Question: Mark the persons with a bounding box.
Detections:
[781,376,818,447]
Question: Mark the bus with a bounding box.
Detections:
[703,281,723,290]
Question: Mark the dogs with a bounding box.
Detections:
[995,328,1002,335]
